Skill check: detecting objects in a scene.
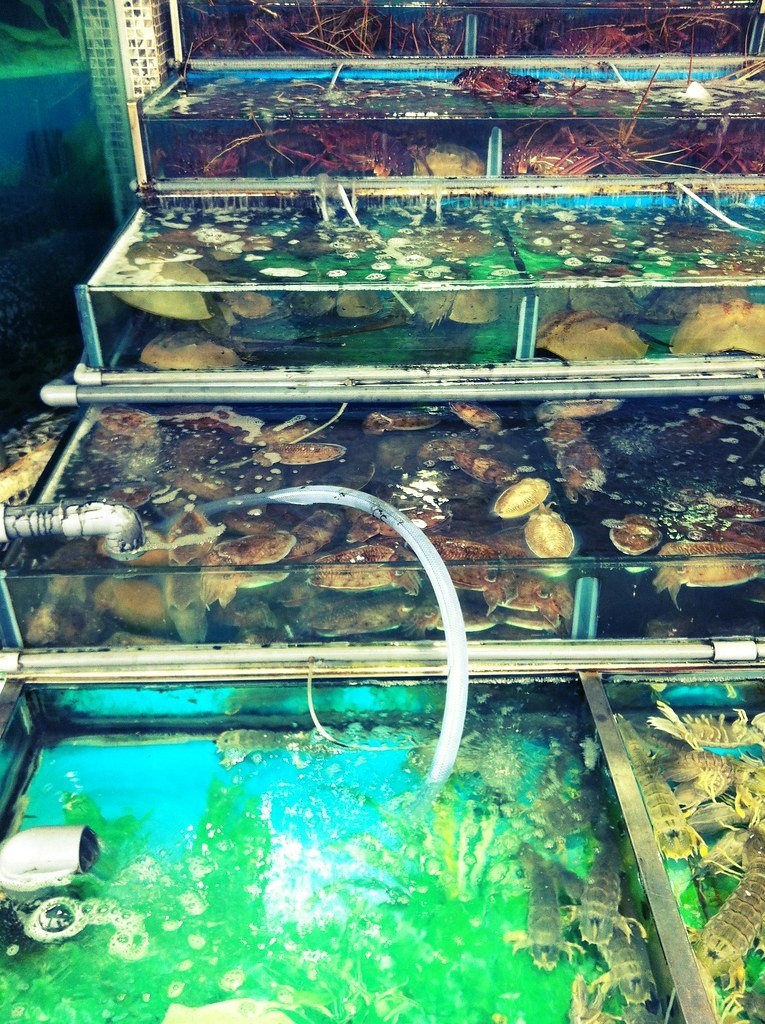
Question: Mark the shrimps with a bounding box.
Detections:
[213,703,765,1023]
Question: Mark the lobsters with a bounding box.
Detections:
[151,0,765,175]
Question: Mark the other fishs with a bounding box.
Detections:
[103,203,503,371]
